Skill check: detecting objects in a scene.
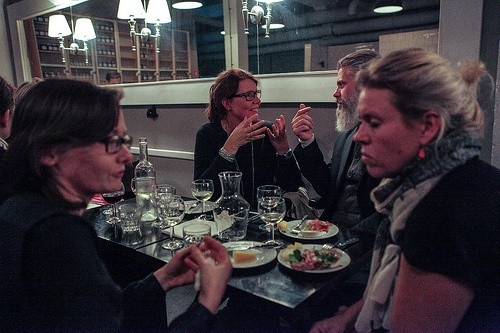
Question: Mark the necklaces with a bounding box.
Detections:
[224,117,255,210]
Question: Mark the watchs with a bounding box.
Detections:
[275,149,292,159]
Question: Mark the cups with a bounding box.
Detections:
[118,203,142,232]
[182,224,211,247]
[131,178,136,195]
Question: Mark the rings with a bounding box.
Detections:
[275,137,279,140]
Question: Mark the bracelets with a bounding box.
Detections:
[219,147,234,162]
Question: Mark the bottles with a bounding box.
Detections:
[134,139,157,222]
[212,172,250,241]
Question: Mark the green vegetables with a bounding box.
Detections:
[289,248,340,269]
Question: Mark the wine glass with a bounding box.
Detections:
[257,186,281,231]
[257,195,286,249]
[101,183,124,224]
[191,179,214,221]
[154,183,185,250]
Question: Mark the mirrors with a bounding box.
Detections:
[16,0,442,87]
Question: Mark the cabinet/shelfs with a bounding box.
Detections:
[23,10,193,84]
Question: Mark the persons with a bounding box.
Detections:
[105,71,121,84]
[0,51,381,333]
[309,48,500,333]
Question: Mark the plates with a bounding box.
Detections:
[184,200,215,215]
[277,244,351,273]
[222,241,277,268]
[280,220,339,240]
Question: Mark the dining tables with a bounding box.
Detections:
[82,192,376,333]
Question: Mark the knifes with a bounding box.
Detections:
[227,242,280,251]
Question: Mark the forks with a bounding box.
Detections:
[290,214,308,234]
[320,238,359,252]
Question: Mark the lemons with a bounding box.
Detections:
[234,252,258,264]
[278,220,288,231]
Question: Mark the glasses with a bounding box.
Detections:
[95,135,132,154]
[227,90,261,102]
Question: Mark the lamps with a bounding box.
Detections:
[172,0,203,10]
[74,18,97,64]
[48,14,77,62]
[145,0,172,53]
[372,0,404,13]
[259,0,285,39]
[117,0,148,51]
